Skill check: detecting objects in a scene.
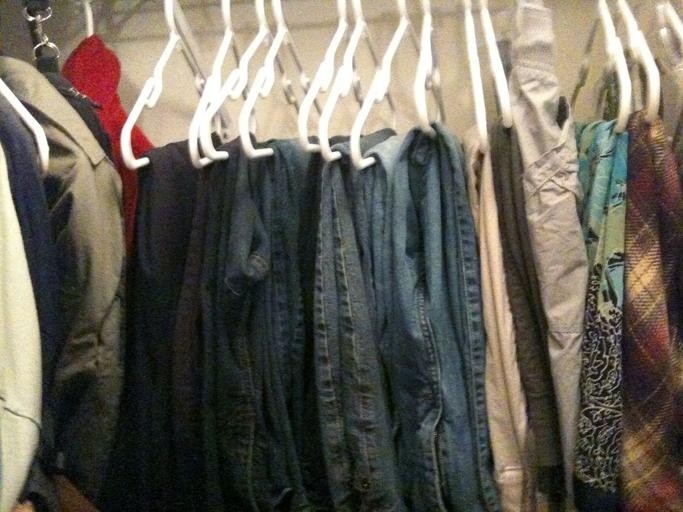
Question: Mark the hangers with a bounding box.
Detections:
[0,74,49,182]
